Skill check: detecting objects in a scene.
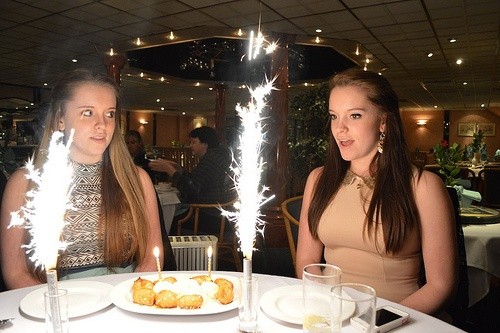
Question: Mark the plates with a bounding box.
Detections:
[148,159,176,164]
[110,274,240,315]
[158,189,172,192]
[20,280,114,318]
[260,284,356,324]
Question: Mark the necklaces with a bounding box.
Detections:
[342,170,377,191]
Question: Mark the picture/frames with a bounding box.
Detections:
[457,121,496,137]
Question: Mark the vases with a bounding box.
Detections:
[473,153,481,162]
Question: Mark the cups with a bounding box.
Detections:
[330,283,377,333]
[302,264,342,333]
[158,182,172,189]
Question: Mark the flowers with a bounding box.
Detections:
[435,140,464,186]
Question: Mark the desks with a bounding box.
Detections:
[155,185,182,236]
[461,162,500,177]
[460,222,500,308]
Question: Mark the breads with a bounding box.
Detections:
[131,275,234,309]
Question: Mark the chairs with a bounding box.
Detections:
[281,165,500,278]
[175,197,243,271]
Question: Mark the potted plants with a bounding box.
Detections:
[471,130,489,153]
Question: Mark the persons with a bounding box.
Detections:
[124,129,158,185]
[294,70,458,314]
[0,69,163,288]
[28,118,42,145]
[148,126,236,235]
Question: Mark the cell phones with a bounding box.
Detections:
[350,305,411,333]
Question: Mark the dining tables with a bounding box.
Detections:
[0,271,465,333]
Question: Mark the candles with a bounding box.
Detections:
[154,246,162,281]
[208,246,212,280]
